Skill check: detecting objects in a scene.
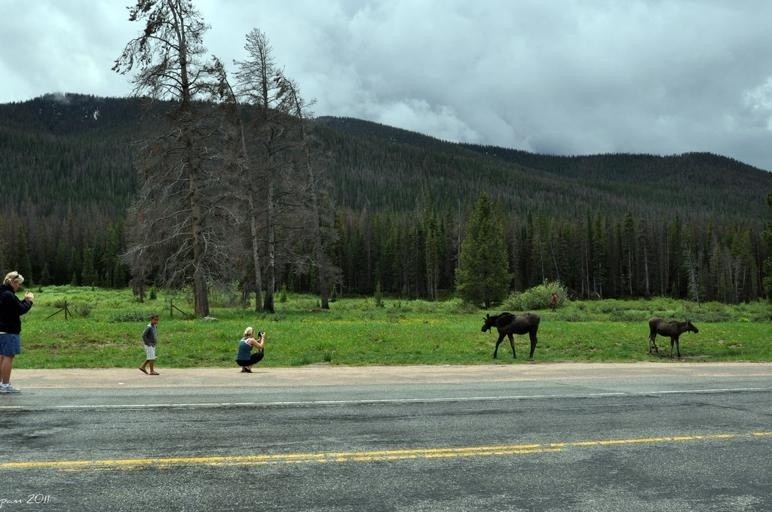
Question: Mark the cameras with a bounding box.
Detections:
[258,331,264,336]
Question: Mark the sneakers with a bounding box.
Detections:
[241,368,252,372]
[139,367,160,375]
[0,384,20,393]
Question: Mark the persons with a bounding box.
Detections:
[235,326,266,373]
[138,313,161,375]
[0,270,34,393]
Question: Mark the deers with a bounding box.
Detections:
[648,316,699,359]
[480,312,541,359]
